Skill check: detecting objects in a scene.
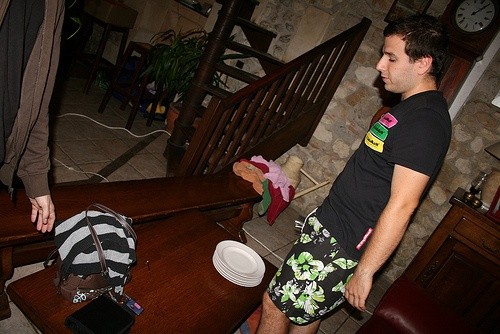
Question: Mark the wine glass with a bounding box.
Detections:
[470,171,489,194]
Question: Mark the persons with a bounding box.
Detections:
[0,0,65,234]
[256,14,453,334]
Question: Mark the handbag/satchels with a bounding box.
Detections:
[65,293,135,334]
[54,203,137,301]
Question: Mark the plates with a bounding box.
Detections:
[212,240,265,288]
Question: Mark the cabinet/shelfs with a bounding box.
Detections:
[403,187,500,327]
[104,0,223,98]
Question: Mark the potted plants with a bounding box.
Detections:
[136,27,236,149]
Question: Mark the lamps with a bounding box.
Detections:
[480,142,500,211]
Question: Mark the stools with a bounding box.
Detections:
[355,275,477,334]
[99,42,167,131]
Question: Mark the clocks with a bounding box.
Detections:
[438,0,500,61]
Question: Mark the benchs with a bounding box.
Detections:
[0,172,263,321]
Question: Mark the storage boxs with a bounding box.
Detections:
[83,0,138,28]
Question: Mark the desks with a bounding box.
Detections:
[63,12,129,96]
[6,207,278,334]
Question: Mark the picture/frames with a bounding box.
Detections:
[383,0,433,24]
